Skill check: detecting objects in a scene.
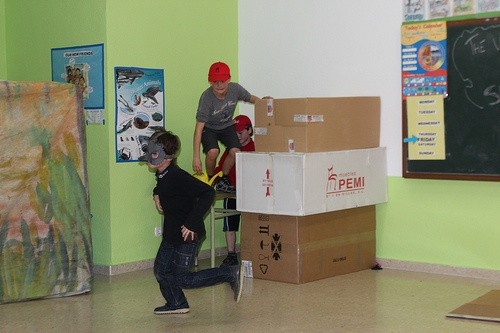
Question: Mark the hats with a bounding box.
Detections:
[208,61,231,82]
[233,114,252,131]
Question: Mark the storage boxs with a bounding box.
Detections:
[236,97,387,284]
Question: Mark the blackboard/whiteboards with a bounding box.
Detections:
[400,11,500,183]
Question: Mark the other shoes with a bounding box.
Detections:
[228,263,245,304]
[216,179,231,193]
[220,252,240,267]
[153,302,190,314]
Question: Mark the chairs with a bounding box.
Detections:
[201,192,239,267]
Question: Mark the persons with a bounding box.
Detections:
[215,115,255,265]
[192,62,274,193]
[67,67,85,87]
[147,131,244,313]
